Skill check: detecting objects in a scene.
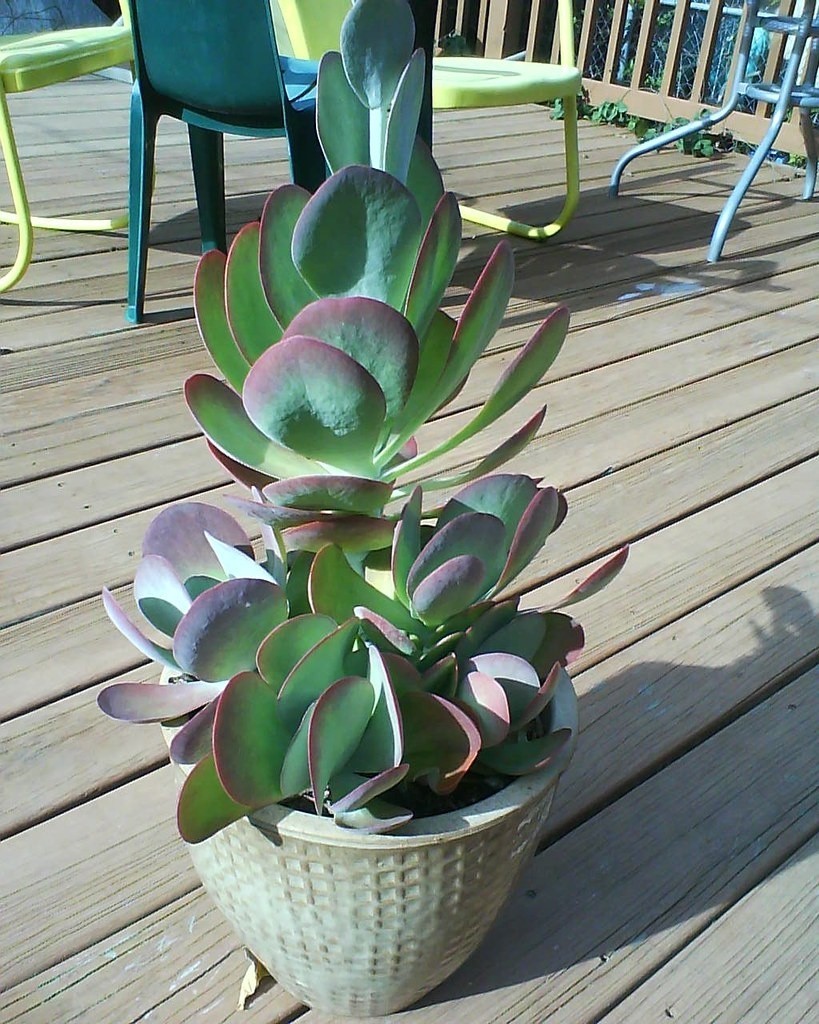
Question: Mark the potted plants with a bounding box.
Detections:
[86,0,632,1015]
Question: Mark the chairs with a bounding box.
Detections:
[0,0,819,330]
